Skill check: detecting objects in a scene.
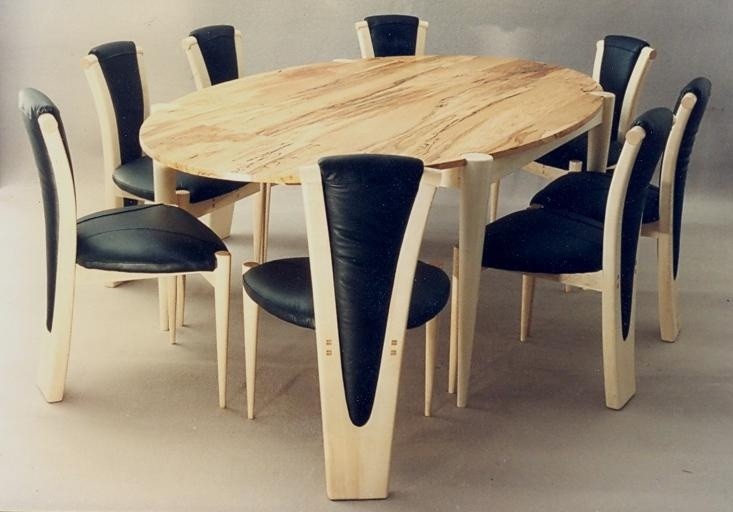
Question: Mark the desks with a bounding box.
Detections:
[138,55,617,408]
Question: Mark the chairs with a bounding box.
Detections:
[18,86,231,409]
[527,77,712,342]
[185,25,280,263]
[81,41,266,326]
[490,35,657,223]
[448,107,677,410]
[242,154,451,500]
[355,15,429,59]
